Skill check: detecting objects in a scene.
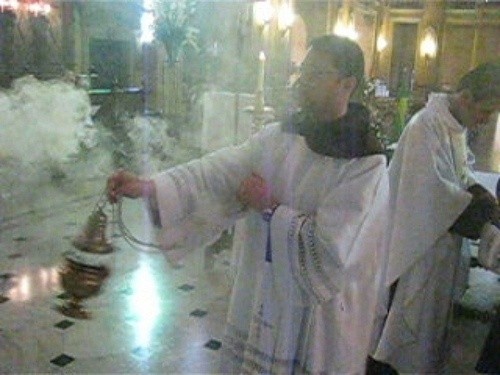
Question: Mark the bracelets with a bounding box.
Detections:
[262,202,279,221]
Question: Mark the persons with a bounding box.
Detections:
[370,63,500,375]
[100,34,390,375]
